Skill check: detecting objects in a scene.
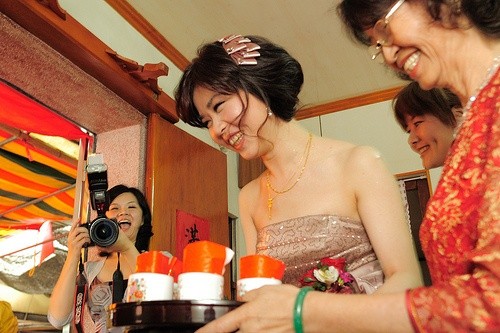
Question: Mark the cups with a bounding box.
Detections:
[177,272,225,301]
[126,273,173,300]
[236,277,284,301]
[173,283,177,300]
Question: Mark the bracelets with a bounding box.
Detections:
[293,286,313,333]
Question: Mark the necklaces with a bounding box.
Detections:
[268,137,313,193]
[267,136,311,219]
[453,54,500,136]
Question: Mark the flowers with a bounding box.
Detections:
[300,257,353,295]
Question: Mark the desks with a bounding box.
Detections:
[18,320,59,333]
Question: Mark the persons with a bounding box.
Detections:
[195,0,500,333]
[47,185,153,333]
[392,81,461,168]
[176,34,424,297]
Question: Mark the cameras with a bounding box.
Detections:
[77,154,122,248]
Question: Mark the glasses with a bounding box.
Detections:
[366,0,404,64]
